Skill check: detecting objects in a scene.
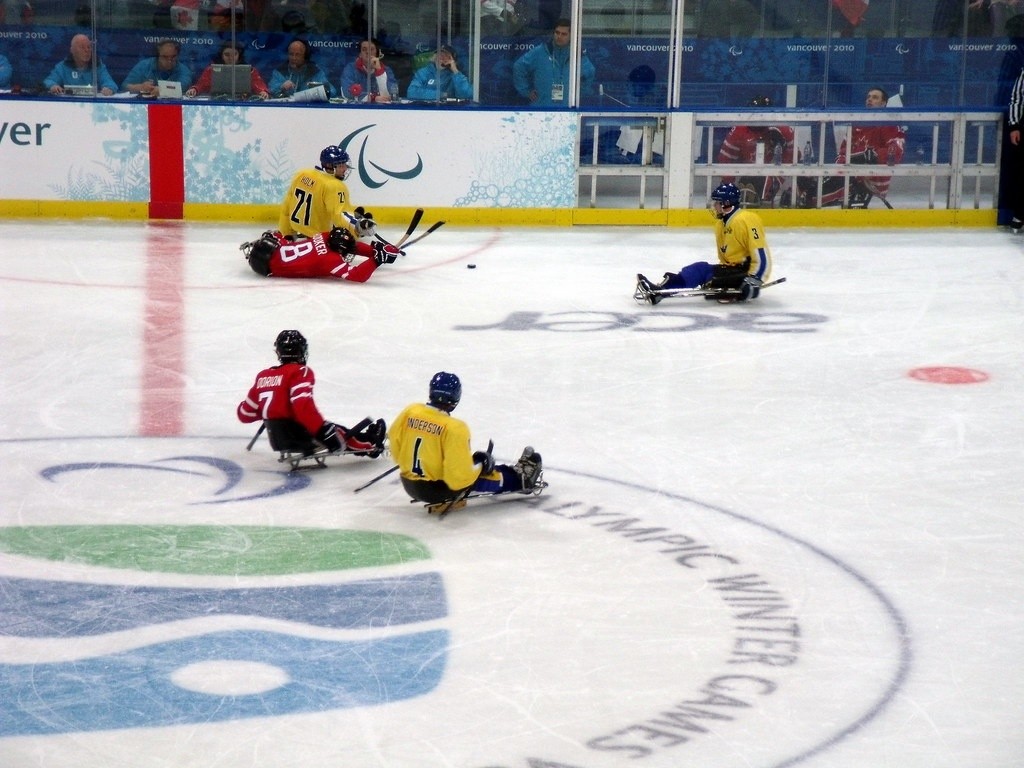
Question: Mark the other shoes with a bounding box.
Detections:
[1010,220,1023,234]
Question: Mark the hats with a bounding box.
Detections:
[434,44,457,61]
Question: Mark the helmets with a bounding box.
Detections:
[705,182,742,219]
[320,145,352,181]
[274,330,309,365]
[745,94,772,107]
[328,228,356,262]
[428,372,461,413]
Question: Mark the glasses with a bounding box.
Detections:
[287,52,304,58]
[159,54,178,61]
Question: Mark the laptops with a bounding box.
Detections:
[211,63,253,96]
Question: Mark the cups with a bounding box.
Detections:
[786,84,796,108]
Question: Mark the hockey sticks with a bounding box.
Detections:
[343,211,406,256]
[760,277,786,290]
[395,209,446,250]
[246,422,266,451]
[438,439,494,520]
[354,464,399,493]
[343,416,374,441]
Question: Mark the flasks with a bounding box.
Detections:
[864,146,873,164]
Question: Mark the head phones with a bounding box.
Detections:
[355,38,381,58]
[287,40,311,63]
[154,40,182,58]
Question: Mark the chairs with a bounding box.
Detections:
[379,53,415,98]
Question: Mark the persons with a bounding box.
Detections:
[279,145,378,242]
[513,16,601,108]
[388,371,542,503]
[781,88,906,211]
[637,182,772,306]
[718,93,802,207]
[0,0,522,105]
[930,0,1024,234]
[237,330,386,458]
[239,226,399,283]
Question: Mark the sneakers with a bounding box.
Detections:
[518,451,547,495]
[353,418,375,458]
[371,418,387,459]
[636,273,664,306]
[511,446,534,474]
[354,206,378,237]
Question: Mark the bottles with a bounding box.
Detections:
[886,141,896,167]
[804,141,812,166]
[773,142,782,167]
[755,137,765,168]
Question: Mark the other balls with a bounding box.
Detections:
[467,264,476,268]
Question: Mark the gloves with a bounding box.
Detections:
[370,242,398,266]
[849,150,875,165]
[735,276,764,302]
[316,420,343,455]
[473,451,496,476]
